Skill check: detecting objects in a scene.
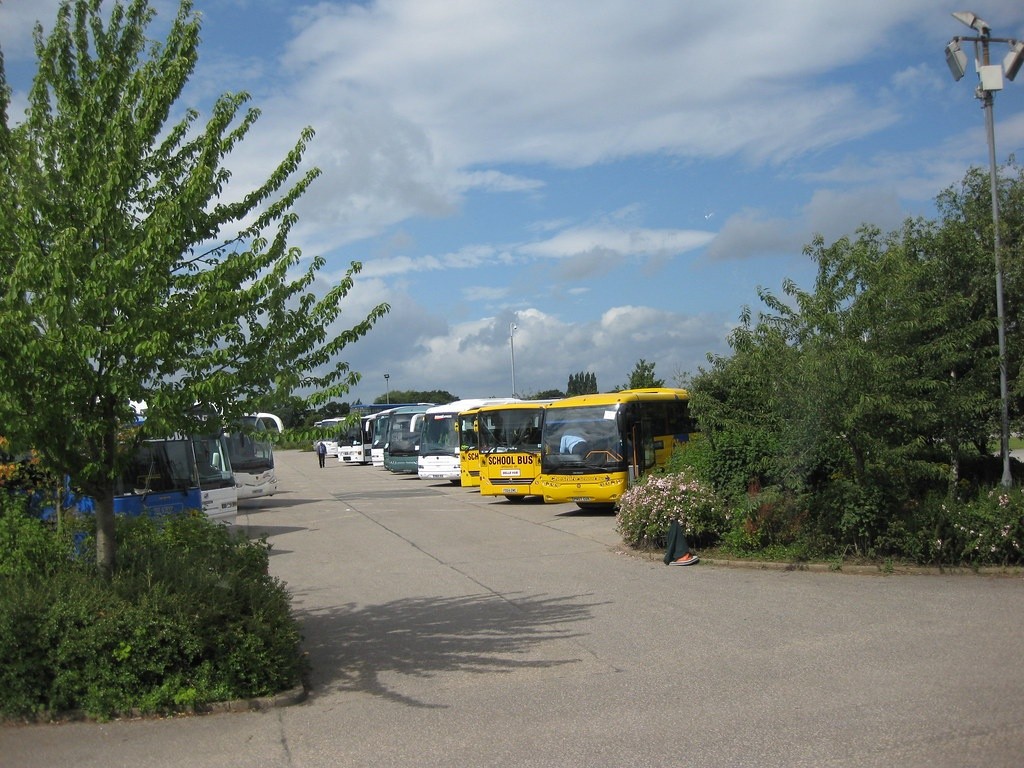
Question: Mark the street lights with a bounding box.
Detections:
[383,373,390,404]
[946,12,1023,487]
[510,322,519,396]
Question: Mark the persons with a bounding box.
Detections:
[167,448,194,480]
[317,442,327,469]
[559,428,590,457]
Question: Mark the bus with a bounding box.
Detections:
[539,388,699,509]
[29,408,285,550]
[313,403,437,475]
[455,410,482,487]
[476,402,547,504]
[409,397,521,485]
